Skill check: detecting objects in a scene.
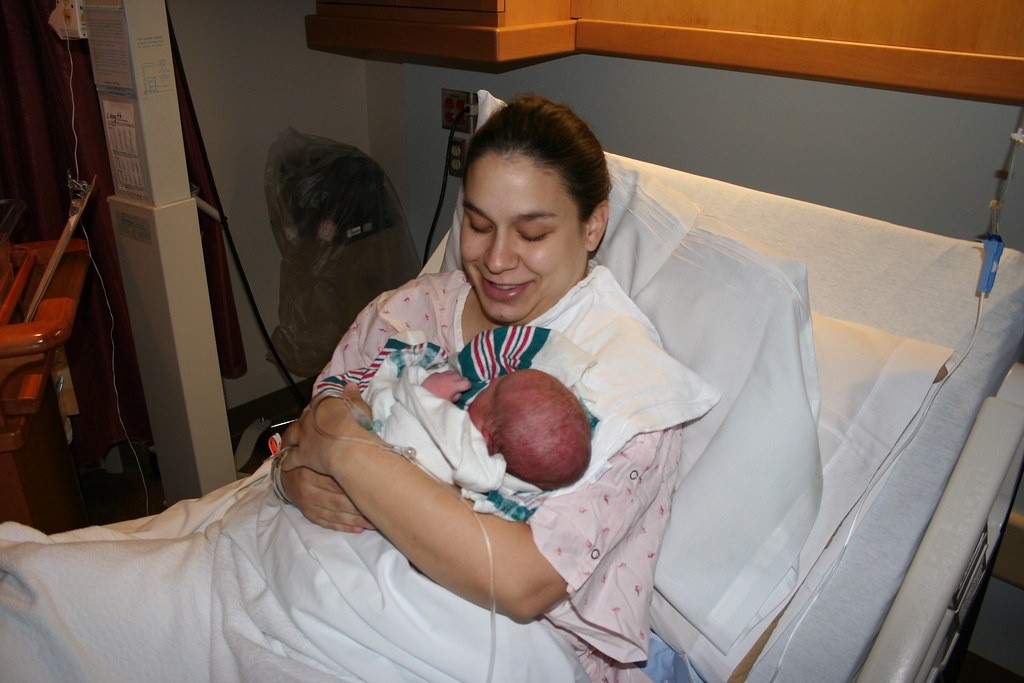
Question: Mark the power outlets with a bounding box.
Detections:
[449,137,466,178]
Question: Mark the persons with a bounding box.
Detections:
[360,370,601,499]
[269,95,683,683]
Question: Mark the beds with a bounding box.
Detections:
[1,91,1024,683]
